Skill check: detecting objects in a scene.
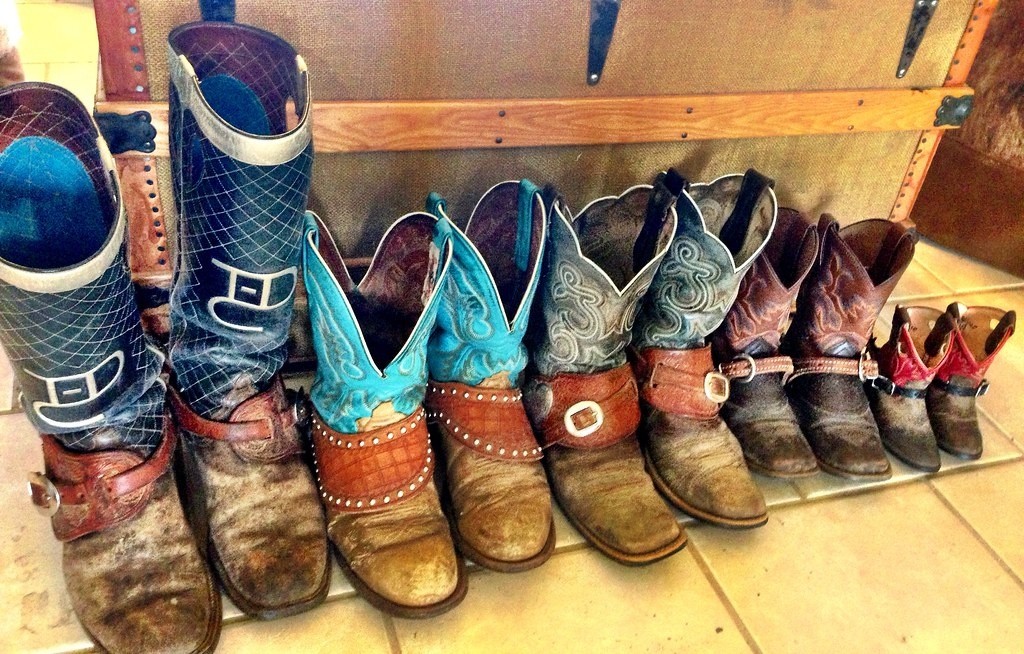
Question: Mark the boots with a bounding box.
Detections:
[705,205,819,478]
[628,164,778,529]
[426,180,556,575]
[866,303,956,473]
[926,301,1016,462]
[164,22,333,622]
[525,181,688,566]
[783,213,920,484]
[302,210,469,620]
[0,82,222,654]
